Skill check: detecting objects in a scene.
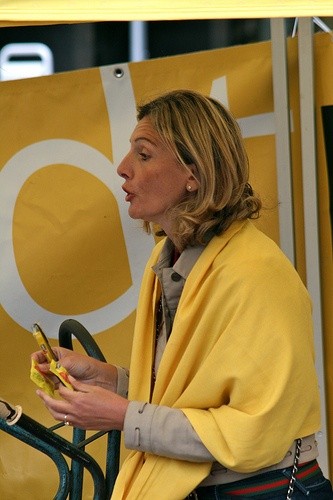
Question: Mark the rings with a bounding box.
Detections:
[64,414,71,426]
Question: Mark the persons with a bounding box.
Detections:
[31,89,333,500]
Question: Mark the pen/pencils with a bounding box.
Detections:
[31,323,58,363]
[282,468,309,495]
